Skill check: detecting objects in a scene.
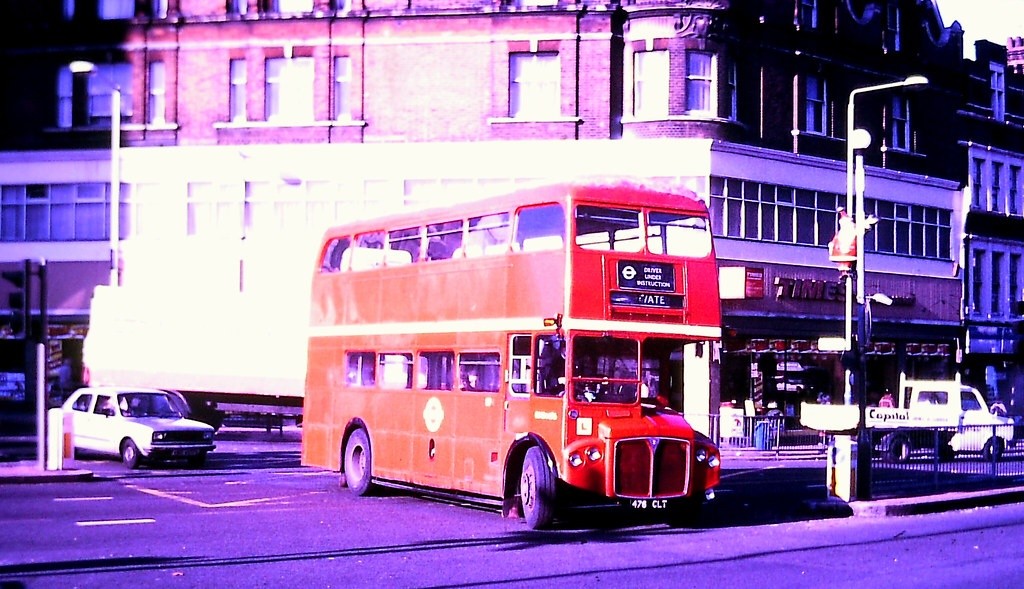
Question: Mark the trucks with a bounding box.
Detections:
[801,373,1014,465]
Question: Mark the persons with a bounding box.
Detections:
[877,387,896,408]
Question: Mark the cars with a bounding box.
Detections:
[51,388,216,468]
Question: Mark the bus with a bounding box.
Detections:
[298,177,725,530]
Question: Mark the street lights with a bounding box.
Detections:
[843,72,930,409]
[68,56,124,392]
